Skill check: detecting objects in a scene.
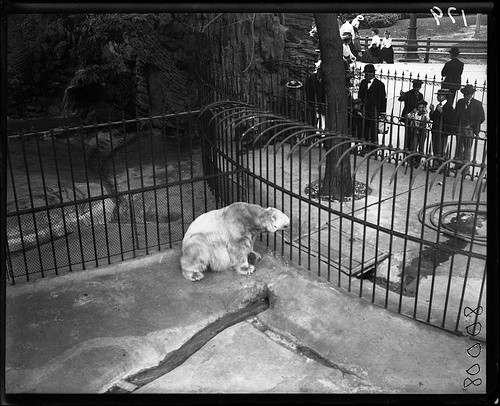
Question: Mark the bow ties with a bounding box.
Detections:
[464,101,469,108]
[365,80,371,83]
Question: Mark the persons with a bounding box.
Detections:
[357,63,387,155]
[441,47,464,105]
[452,84,486,174]
[339,14,355,41]
[342,36,356,66]
[398,79,424,155]
[306,64,325,112]
[381,30,394,63]
[406,101,430,167]
[367,28,381,63]
[351,15,365,51]
[428,88,454,168]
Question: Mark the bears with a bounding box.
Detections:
[180,201,290,282]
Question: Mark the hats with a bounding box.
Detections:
[384,31,391,35]
[415,101,427,107]
[362,65,376,72]
[371,28,379,34]
[412,80,424,87]
[357,15,364,21]
[461,85,476,94]
[434,89,452,95]
[448,48,461,53]
